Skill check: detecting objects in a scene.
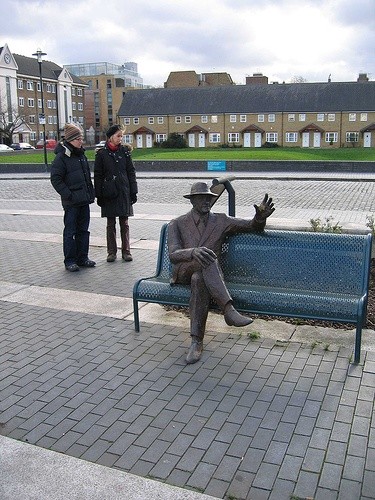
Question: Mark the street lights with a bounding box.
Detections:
[31,47,48,173]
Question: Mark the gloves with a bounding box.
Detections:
[96,196,103,207]
[130,193,137,204]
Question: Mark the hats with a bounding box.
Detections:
[64,123,83,142]
[105,125,122,139]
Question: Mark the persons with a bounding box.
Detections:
[51,122,95,272]
[94,125,138,262]
[167,182,275,365]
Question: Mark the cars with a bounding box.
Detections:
[36,138,57,149]
[95,141,106,147]
[0,144,15,151]
[10,143,35,150]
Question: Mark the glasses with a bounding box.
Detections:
[75,137,84,141]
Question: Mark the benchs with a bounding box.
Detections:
[133,222,373,363]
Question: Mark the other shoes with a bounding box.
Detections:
[64,262,79,272]
[106,253,116,262]
[121,253,132,261]
[77,259,95,267]
